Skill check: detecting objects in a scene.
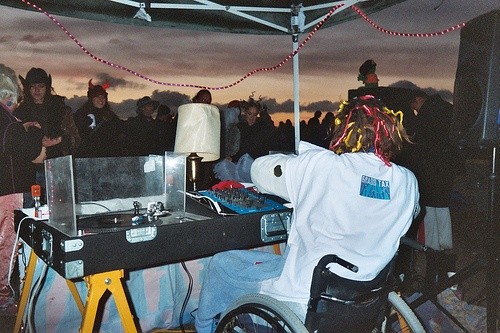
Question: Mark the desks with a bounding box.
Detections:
[13,186,293,333]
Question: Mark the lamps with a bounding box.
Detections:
[174,102,221,191]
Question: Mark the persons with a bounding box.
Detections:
[194,93,423,333]
[72,78,125,157]
[118,89,457,191]
[0,63,47,310]
[12,66,81,207]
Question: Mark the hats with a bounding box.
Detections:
[88,78,109,102]
[136,96,159,113]
[18,67,52,89]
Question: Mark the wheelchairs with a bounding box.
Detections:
[212,236,428,333]
